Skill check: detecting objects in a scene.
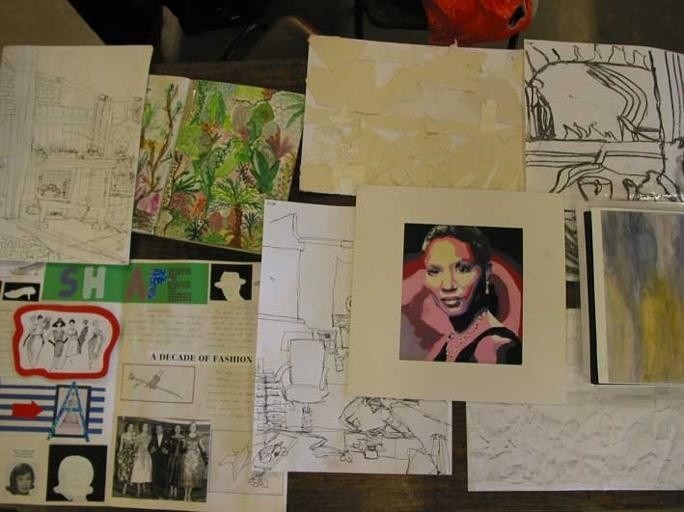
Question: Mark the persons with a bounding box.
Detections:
[117,422,138,494]
[179,421,207,501]
[5,462,36,495]
[130,422,154,496]
[165,424,186,498]
[148,423,177,499]
[413,226,521,363]
[24,314,104,370]
